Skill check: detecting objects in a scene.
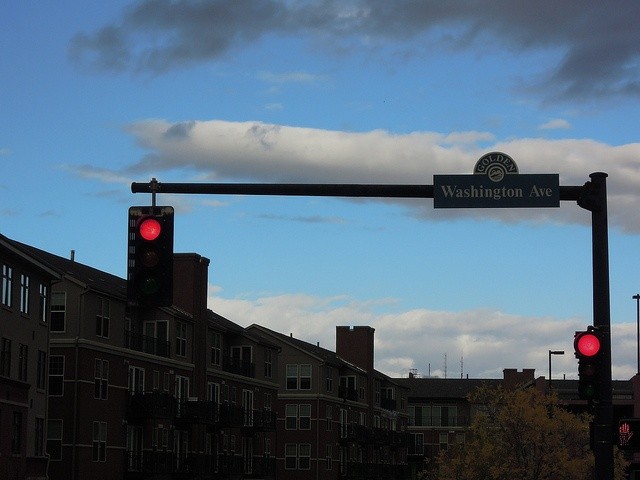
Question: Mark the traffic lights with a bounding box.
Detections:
[573,326,612,401]
[128,206,174,307]
